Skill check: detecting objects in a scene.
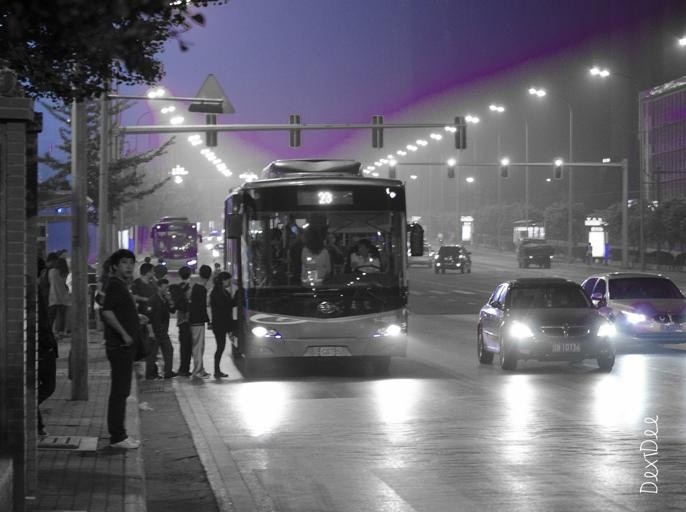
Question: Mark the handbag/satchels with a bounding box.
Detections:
[131,324,151,360]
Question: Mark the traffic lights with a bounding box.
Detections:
[388,159,396,178]
[447,157,455,177]
[500,156,511,179]
[553,157,564,181]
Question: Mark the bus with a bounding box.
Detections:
[222,157,423,383]
[150,215,203,276]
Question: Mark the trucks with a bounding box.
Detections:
[514,241,554,267]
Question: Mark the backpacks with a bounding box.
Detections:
[169,283,189,312]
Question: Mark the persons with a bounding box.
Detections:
[245,223,380,287]
[98,248,153,451]
[30,245,71,434]
[132,255,240,380]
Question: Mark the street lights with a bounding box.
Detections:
[363,114,481,240]
[591,64,647,271]
[526,86,576,268]
[487,103,505,257]
[107,84,224,258]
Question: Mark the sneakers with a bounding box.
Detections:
[145,370,228,380]
[110,435,140,449]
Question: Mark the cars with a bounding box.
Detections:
[432,243,470,274]
[476,276,624,372]
[583,272,686,355]
[405,244,431,268]
